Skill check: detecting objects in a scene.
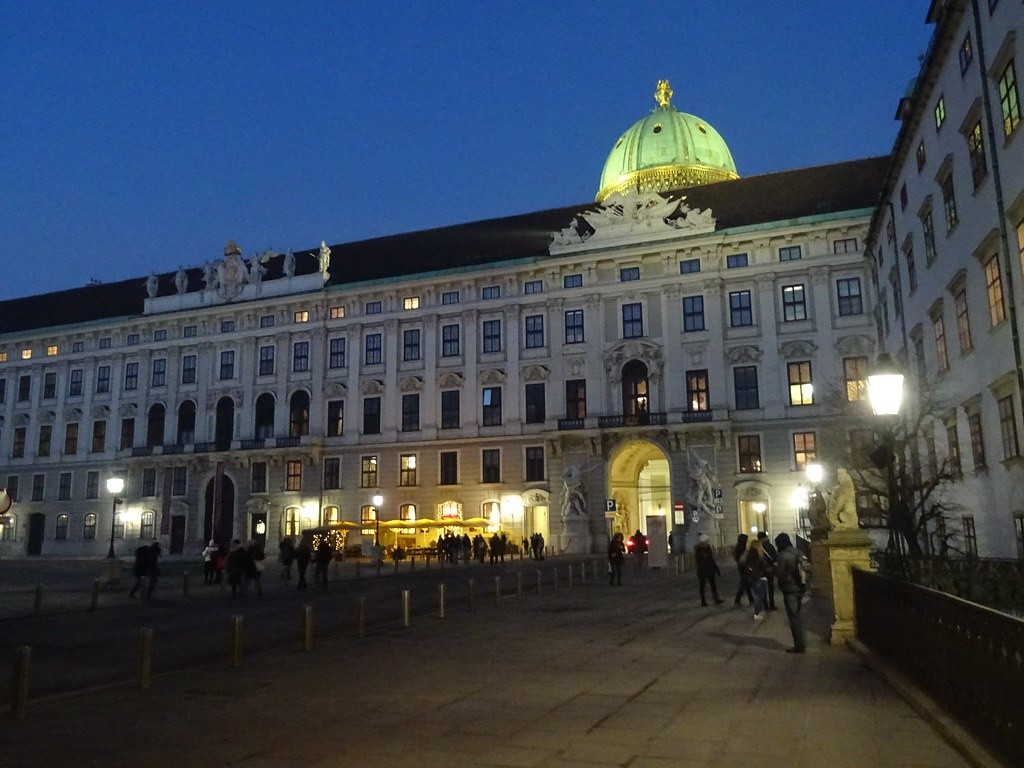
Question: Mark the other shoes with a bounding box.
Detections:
[715,600,724,604]
[764,605,777,611]
[608,582,622,587]
[701,601,707,606]
[786,646,805,653]
[749,601,754,606]
[735,601,742,606]
[754,608,762,615]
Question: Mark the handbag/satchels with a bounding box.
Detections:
[744,560,767,580]
[607,561,614,575]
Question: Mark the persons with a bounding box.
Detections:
[283,248,296,277]
[201,261,218,289]
[693,534,725,606]
[632,530,646,569]
[246,251,266,282]
[437,532,507,565]
[134,541,160,590]
[523,537,528,555]
[202,539,266,597]
[142,271,158,298]
[560,459,602,519]
[319,239,331,272]
[609,532,625,587]
[688,464,713,511]
[531,532,544,560]
[668,531,672,550]
[171,266,188,294]
[278,530,332,591]
[733,532,806,654]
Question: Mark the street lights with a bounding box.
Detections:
[372,494,383,547]
[106,478,125,560]
[862,358,907,580]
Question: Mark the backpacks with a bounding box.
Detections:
[797,553,813,586]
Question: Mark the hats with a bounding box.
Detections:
[700,535,709,542]
